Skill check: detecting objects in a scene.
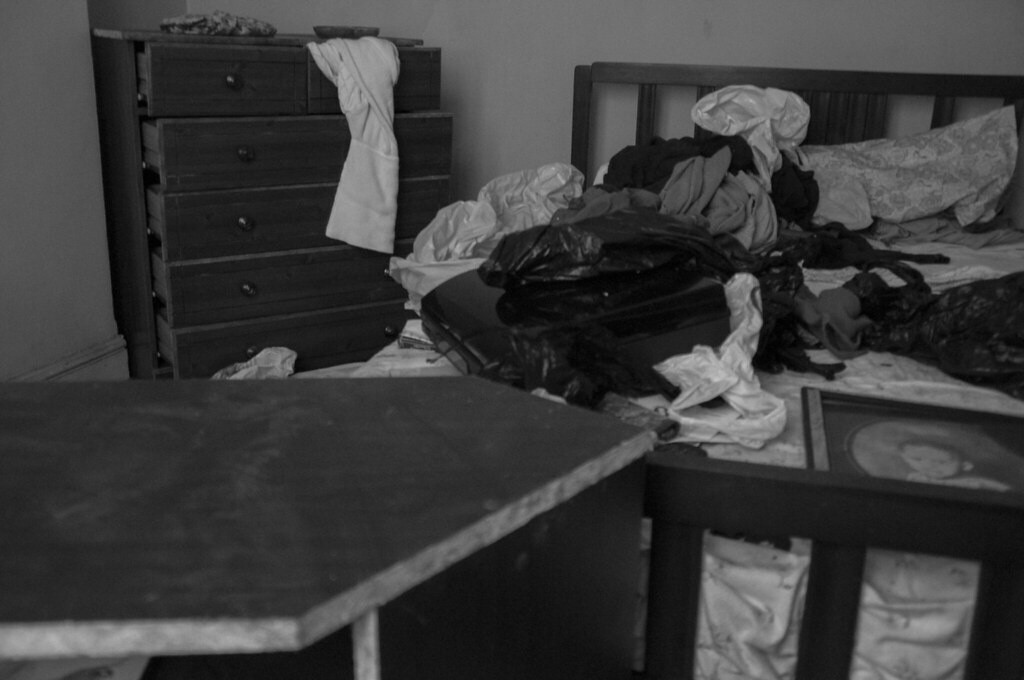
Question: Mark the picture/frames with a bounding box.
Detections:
[799,384,1024,605]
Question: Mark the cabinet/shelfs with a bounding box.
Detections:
[90,28,457,382]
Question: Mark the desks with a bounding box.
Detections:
[0,381,655,680]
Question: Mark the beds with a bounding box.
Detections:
[348,63,1024,680]
